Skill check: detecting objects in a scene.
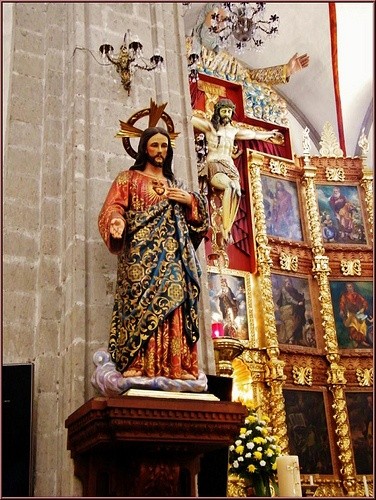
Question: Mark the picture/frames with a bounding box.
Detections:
[270,270,323,353]
[314,181,373,251]
[260,172,310,247]
[328,277,374,357]
[283,385,340,482]
[344,388,373,482]
[206,266,256,349]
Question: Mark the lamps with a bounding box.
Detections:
[209,3,280,54]
[212,324,224,336]
[99,29,164,97]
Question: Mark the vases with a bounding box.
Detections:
[253,475,271,497]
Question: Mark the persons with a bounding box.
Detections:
[97,126,210,380]
[193,98,279,248]
[182,23,310,124]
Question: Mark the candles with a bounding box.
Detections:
[276,455,301,497]
[363,476,369,497]
[310,475,314,485]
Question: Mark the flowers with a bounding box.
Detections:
[229,414,280,488]
